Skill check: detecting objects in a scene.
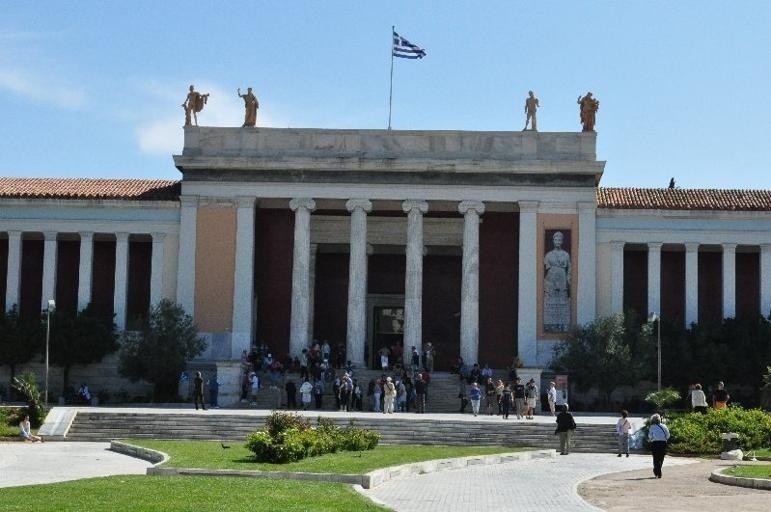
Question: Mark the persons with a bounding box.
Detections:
[542,231,572,333]
[615,409,631,457]
[238,87,259,127]
[555,403,576,455]
[577,91,599,131]
[691,384,708,414]
[19,414,41,443]
[77,383,91,404]
[208,375,221,409]
[712,381,730,409]
[647,414,670,479]
[521,90,540,131]
[193,371,205,410]
[181,84,210,126]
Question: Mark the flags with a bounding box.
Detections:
[391,31,427,60]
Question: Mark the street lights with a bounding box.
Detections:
[41,298,57,405]
[646,310,663,392]
